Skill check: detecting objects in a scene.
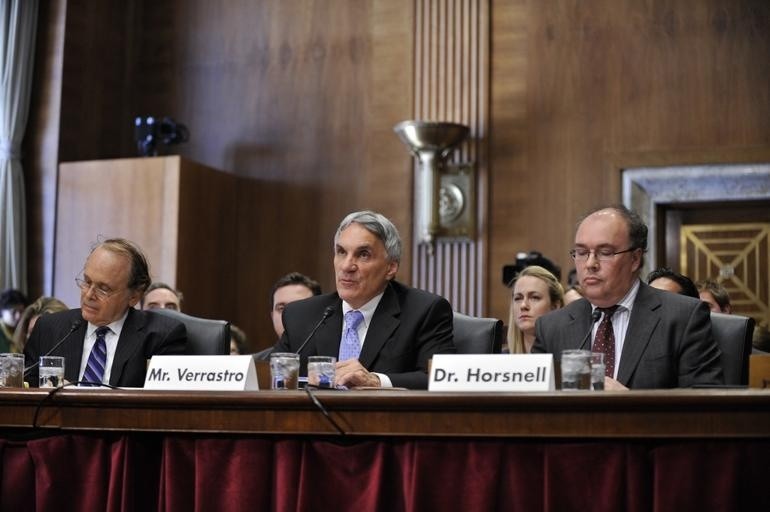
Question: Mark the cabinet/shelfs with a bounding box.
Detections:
[52,156,222,319]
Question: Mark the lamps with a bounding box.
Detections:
[395,119,475,252]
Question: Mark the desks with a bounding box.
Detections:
[0,387,770,512]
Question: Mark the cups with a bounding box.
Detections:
[558,347,608,392]
[38,355,65,390]
[268,351,301,390]
[0,352,26,390]
[307,355,338,390]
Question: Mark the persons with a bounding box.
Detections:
[229,323,249,355]
[527,204,722,392]
[500,265,768,354]
[265,210,456,390]
[250,272,323,363]
[1,238,186,388]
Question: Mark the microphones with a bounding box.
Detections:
[295,306,336,352]
[575,310,602,350]
[25,319,82,378]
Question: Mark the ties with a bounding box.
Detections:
[80,326,110,387]
[336,310,365,362]
[592,305,621,379]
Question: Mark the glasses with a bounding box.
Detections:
[572,244,633,260]
[74,266,131,299]
